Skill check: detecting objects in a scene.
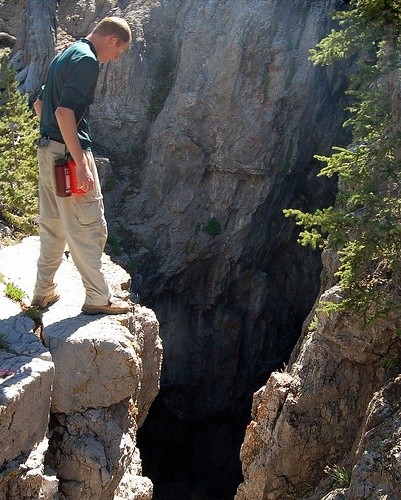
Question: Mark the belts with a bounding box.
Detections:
[41,133,65,144]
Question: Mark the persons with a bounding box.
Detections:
[32,16,135,316]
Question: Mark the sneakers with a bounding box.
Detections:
[82,298,129,314]
[31,291,60,307]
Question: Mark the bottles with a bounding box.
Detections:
[64,152,88,194]
[54,159,72,197]
